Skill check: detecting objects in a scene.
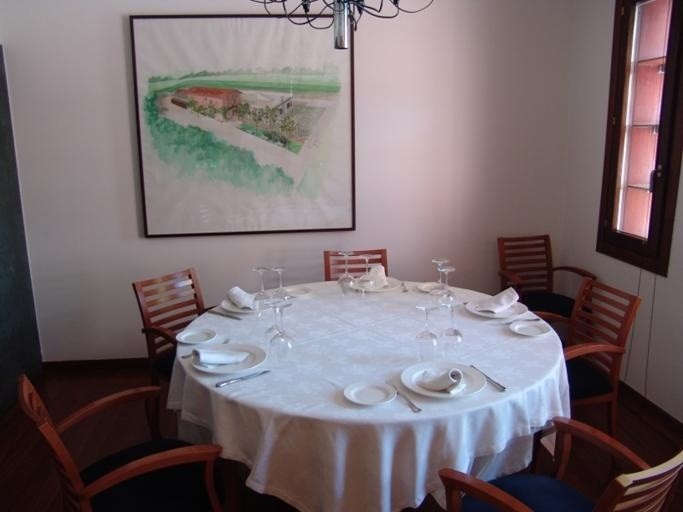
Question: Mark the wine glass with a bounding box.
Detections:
[250,250,465,367]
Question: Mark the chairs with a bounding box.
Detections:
[529,274,643,477]
[439,413,683,511]
[132,267,217,386]
[496,233,603,325]
[17,371,224,511]
[325,248,388,283]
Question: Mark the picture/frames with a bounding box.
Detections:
[128,12,357,237]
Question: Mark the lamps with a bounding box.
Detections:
[256,1,434,54]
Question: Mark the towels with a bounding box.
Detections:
[227,285,257,312]
[191,347,251,368]
[418,365,468,395]
[475,285,521,315]
[353,262,388,291]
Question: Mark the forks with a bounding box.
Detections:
[385,380,423,412]
[180,339,231,362]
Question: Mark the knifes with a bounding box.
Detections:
[216,370,270,388]
[469,365,509,393]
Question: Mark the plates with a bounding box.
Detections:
[400,361,487,398]
[342,380,397,406]
[467,300,529,321]
[508,319,549,337]
[355,274,403,292]
[190,343,266,373]
[222,294,266,315]
[179,327,217,344]
[417,281,444,295]
